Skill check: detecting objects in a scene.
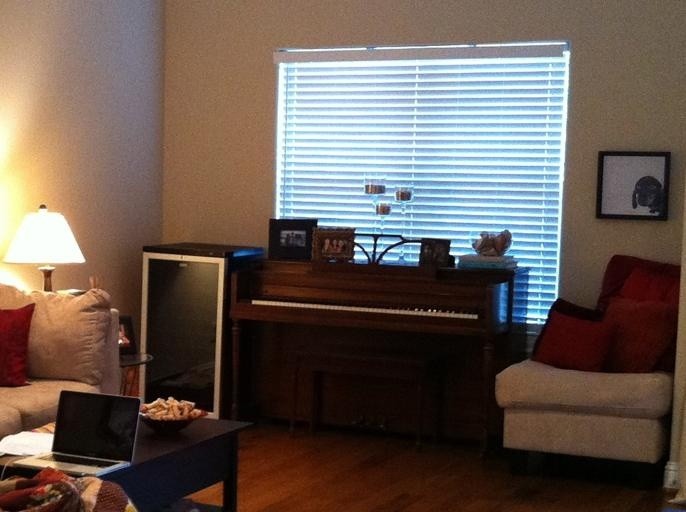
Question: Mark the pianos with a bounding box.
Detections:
[230,254,529,449]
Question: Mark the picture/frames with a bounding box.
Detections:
[117,313,138,356]
[309,226,357,261]
[595,150,672,222]
[266,217,319,261]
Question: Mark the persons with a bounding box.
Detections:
[280,231,351,255]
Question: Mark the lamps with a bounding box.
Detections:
[2,203,87,292]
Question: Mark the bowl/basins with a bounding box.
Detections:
[138,409,208,433]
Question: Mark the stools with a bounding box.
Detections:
[282,347,448,453]
[496,356,675,487]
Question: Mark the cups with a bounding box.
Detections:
[365,179,385,194]
[395,186,413,202]
[376,204,392,215]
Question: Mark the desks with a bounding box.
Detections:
[117,353,155,367]
[0,402,255,510]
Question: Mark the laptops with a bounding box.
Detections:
[12,390,143,478]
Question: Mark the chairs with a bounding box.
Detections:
[0,282,124,441]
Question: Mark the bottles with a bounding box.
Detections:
[117,322,131,358]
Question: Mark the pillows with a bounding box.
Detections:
[532,251,681,373]
[27,286,113,386]
[0,298,39,387]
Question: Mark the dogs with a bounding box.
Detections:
[632,175,665,216]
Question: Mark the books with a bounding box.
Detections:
[457,254,513,264]
[457,261,520,270]
